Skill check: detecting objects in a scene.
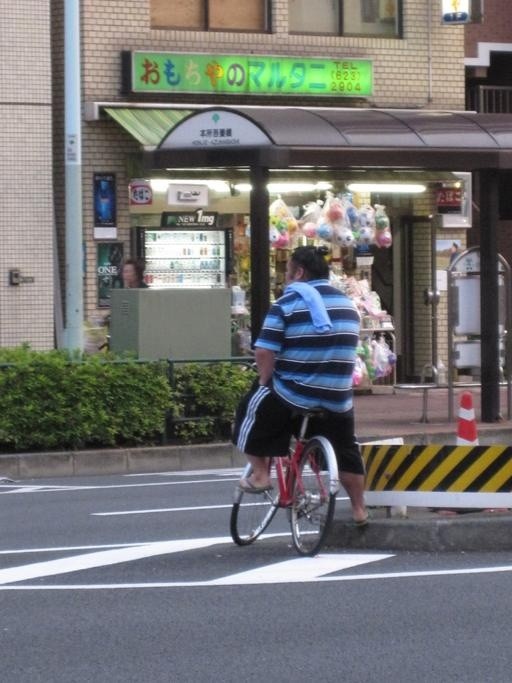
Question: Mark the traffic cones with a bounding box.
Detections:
[455,390,480,447]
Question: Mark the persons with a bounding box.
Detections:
[231,244,378,528]
[121,259,148,287]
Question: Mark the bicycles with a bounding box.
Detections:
[225,322,343,560]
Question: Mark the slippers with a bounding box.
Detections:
[352,508,373,528]
[238,476,273,494]
